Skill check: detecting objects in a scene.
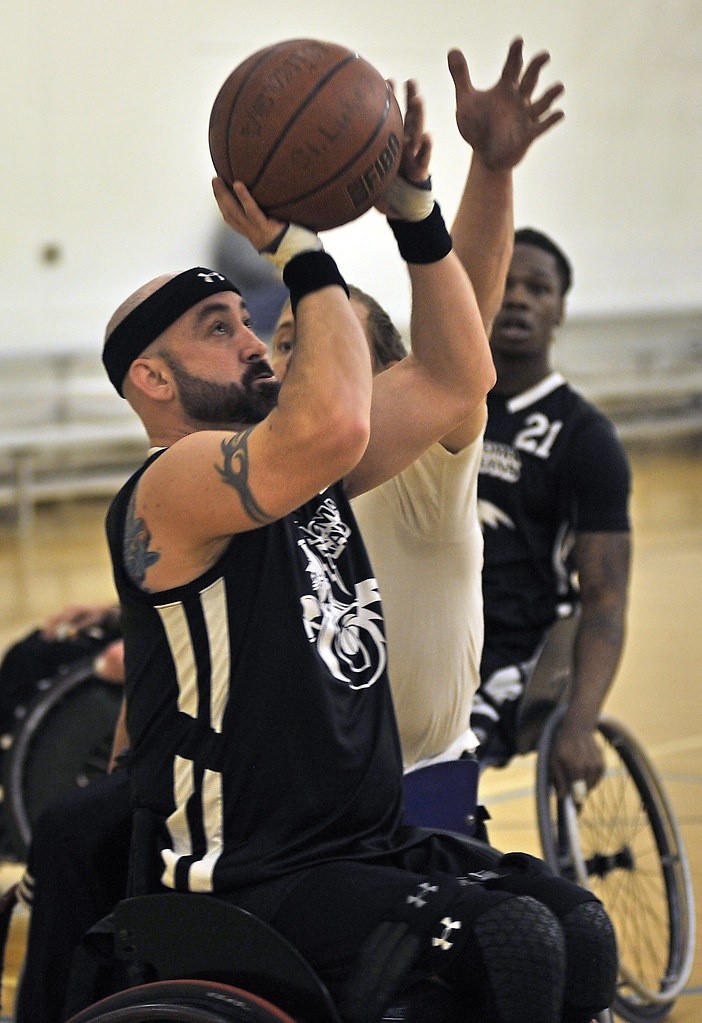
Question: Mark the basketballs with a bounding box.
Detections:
[209,39,406,236]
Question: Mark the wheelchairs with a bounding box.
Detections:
[0,603,694,1023]
[15,764,511,1023]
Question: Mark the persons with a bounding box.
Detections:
[0,35,566,1023]
[103,79,616,1023]
[471,228,634,819]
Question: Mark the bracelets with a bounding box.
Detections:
[283,252,350,313]
[387,199,453,264]
